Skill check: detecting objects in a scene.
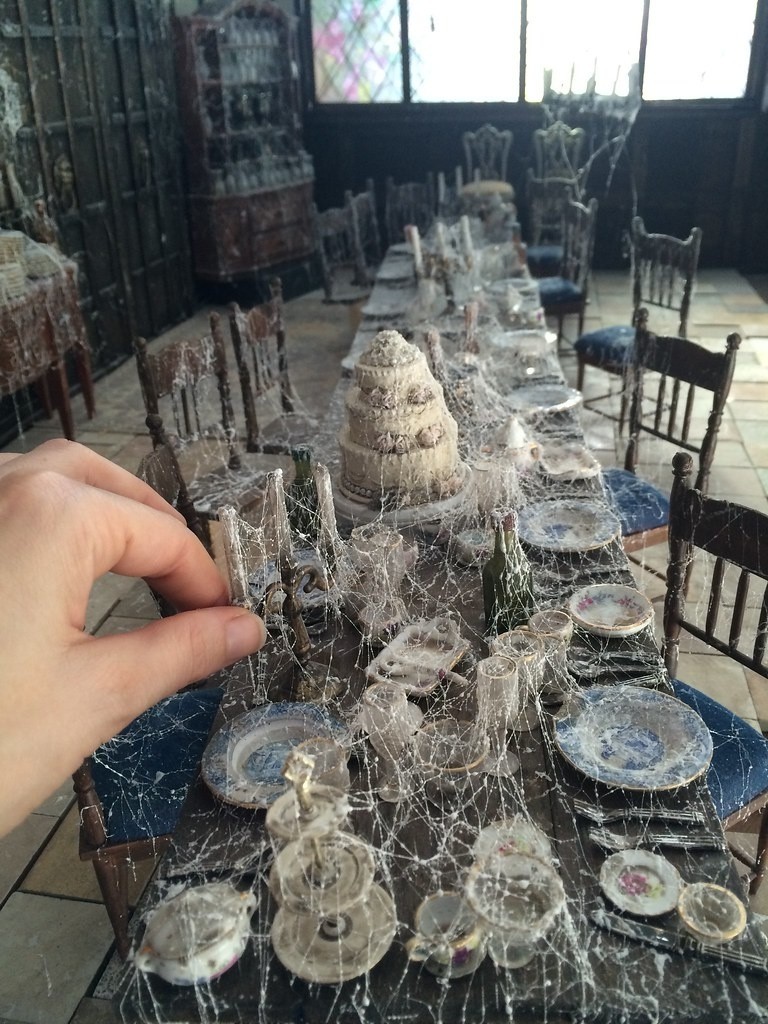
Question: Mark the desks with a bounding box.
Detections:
[113,240,768,1023]
[0,262,98,442]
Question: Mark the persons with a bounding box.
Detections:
[0,438,268,839]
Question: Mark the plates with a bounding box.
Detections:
[0,231,63,300]
[507,385,583,413]
[486,278,537,293]
[493,329,558,350]
[554,685,713,789]
[376,266,416,279]
[365,625,469,697]
[341,351,364,370]
[473,819,552,870]
[358,700,424,736]
[517,499,621,552]
[359,302,406,315]
[202,702,352,806]
[599,849,682,915]
[266,785,397,983]
[250,550,349,612]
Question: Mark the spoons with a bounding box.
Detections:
[379,656,469,688]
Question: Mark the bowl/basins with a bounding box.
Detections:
[569,583,653,638]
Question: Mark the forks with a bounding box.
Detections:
[590,827,725,850]
[574,799,704,823]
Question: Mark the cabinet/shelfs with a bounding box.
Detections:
[172,0,315,299]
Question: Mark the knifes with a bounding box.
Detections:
[591,909,768,973]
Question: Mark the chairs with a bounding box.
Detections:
[136,313,292,549]
[601,307,741,602]
[573,217,703,434]
[309,119,595,358]
[227,277,325,454]
[119,413,215,621]
[73,688,228,963]
[658,451,768,897]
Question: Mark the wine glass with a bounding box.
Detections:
[362,681,415,803]
[477,656,519,777]
[528,610,572,693]
[359,530,404,627]
[417,718,489,810]
[492,630,545,731]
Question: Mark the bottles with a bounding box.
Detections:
[285,447,321,548]
[483,509,533,634]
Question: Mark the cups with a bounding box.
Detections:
[407,890,486,979]
[136,887,257,986]
[679,883,746,945]
[465,854,564,969]
